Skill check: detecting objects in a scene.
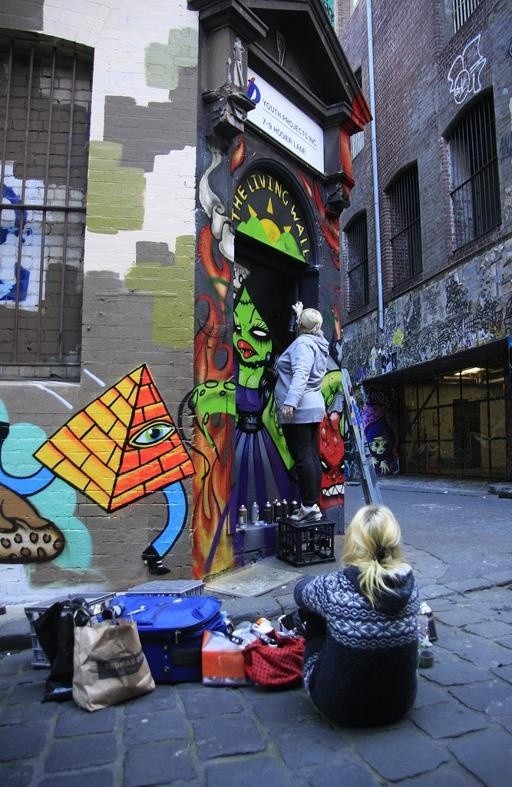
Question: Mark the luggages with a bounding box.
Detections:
[107,593,229,685]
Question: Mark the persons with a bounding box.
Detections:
[294,503,420,730]
[274,302,329,522]
[0,182,32,303]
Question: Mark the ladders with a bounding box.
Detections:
[341,367,383,504]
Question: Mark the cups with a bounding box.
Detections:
[420,650,435,668]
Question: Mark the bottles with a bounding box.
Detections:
[420,601,438,642]
[220,610,234,635]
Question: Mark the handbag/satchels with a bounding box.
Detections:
[29,595,157,714]
[202,613,307,689]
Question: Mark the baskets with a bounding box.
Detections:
[276,514,336,567]
[25,590,116,668]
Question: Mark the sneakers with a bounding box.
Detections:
[286,500,323,523]
